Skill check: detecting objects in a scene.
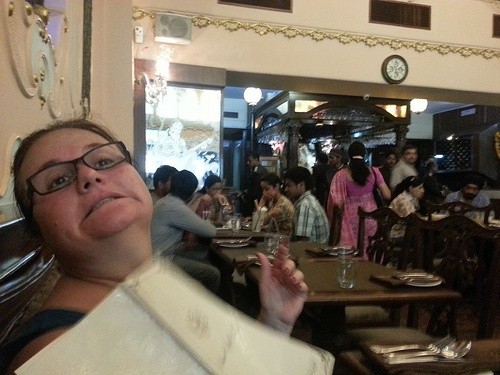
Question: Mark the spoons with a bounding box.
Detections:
[385,340,471,364]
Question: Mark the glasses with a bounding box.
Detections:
[26,141,132,217]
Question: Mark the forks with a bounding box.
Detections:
[369,336,456,353]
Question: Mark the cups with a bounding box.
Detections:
[203,211,211,221]
[229,217,240,229]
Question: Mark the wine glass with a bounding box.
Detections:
[336,244,355,290]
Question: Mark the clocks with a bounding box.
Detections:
[382,55,408,84]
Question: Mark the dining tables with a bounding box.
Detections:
[341,340,500,375]
[216,230,287,241]
[212,241,460,328]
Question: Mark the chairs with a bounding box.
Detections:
[359,199,500,339]
[329,203,345,248]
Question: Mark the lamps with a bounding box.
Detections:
[244,87,262,106]
[410,99,428,114]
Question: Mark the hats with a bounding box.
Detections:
[330,149,344,159]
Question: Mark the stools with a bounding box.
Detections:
[343,305,439,348]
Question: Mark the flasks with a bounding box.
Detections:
[252,210,260,232]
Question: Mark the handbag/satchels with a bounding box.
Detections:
[371,166,386,208]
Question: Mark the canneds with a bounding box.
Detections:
[203,211,211,220]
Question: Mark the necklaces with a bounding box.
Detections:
[333,163,343,171]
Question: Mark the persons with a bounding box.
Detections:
[258,173,294,237]
[312,152,329,214]
[424,158,448,200]
[233,152,270,218]
[284,166,330,246]
[151,165,179,206]
[444,175,495,218]
[378,150,398,186]
[327,141,391,262]
[150,169,221,294]
[191,175,229,220]
[382,176,443,267]
[0,119,308,375]
[327,146,349,247]
[390,145,419,193]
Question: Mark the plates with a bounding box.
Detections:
[403,273,443,287]
[220,243,248,248]
